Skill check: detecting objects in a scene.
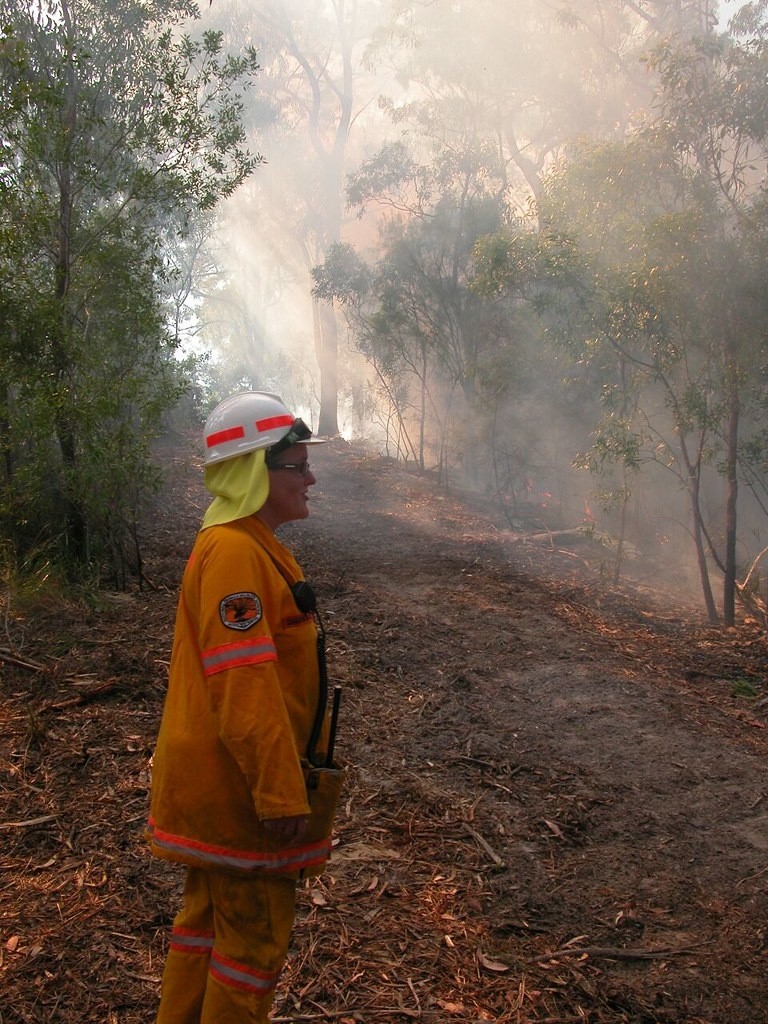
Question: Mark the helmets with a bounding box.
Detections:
[199,391,325,468]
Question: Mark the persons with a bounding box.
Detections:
[151,393,349,1024]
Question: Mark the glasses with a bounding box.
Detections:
[267,462,309,476]
[266,417,312,457]
